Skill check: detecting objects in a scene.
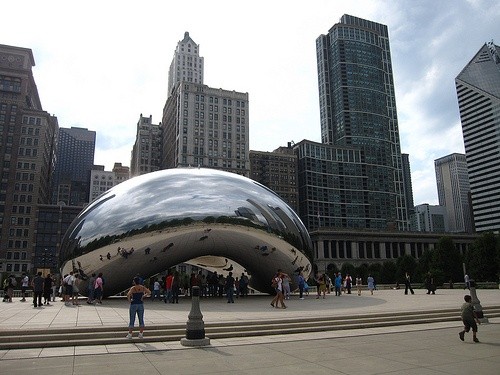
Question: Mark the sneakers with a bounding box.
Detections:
[138,333,143,338]
[459,331,464,341]
[473,338,479,343]
[126,333,132,338]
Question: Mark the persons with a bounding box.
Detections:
[63,218,311,279]
[2,270,457,310]
[459,295,482,343]
[127,278,152,340]
[465,273,471,290]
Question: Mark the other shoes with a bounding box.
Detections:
[4,298,104,308]
[152,297,235,303]
[271,303,274,307]
[284,293,441,300]
[282,307,285,308]
[285,306,288,308]
[275,307,280,308]
